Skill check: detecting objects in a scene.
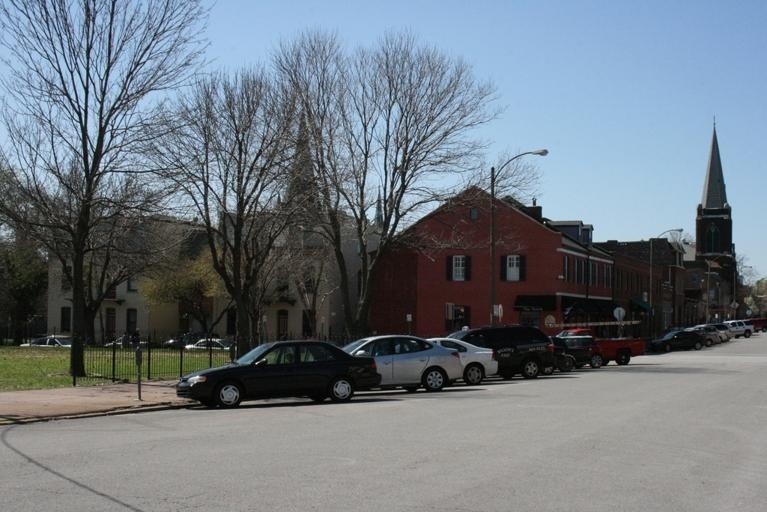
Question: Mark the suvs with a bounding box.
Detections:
[446,325,553,380]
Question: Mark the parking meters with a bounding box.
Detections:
[133,345,145,401]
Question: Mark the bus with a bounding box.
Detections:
[513,293,646,367]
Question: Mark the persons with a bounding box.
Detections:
[120,327,219,349]
[230,334,246,360]
[250,332,259,347]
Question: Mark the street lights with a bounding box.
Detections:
[733,265,753,320]
[488,149,549,328]
[649,227,683,329]
[706,251,734,323]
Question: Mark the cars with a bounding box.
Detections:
[19,335,72,349]
[651,318,767,352]
[424,337,501,386]
[162,331,221,350]
[540,327,605,376]
[103,335,149,350]
[183,338,238,353]
[340,334,464,392]
[175,340,383,409]
